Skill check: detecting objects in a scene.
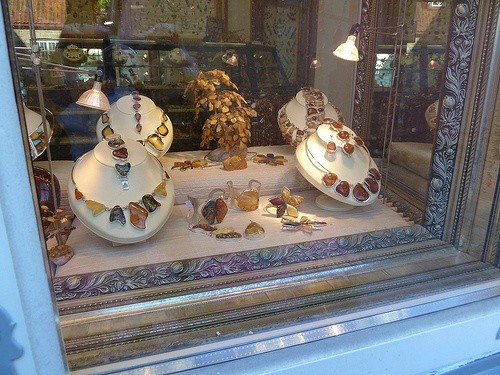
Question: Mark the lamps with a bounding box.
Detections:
[332,23,404,203]
[27,0,110,257]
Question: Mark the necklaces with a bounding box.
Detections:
[27,104,55,160]
[69,149,171,231]
[306,134,381,204]
[64,44,83,58]
[169,48,186,63]
[101,99,168,151]
[278,95,344,147]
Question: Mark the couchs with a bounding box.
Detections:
[388,99,440,181]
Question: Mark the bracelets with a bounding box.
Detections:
[104,132,130,192]
[301,86,326,129]
[322,117,354,156]
[132,91,141,135]
